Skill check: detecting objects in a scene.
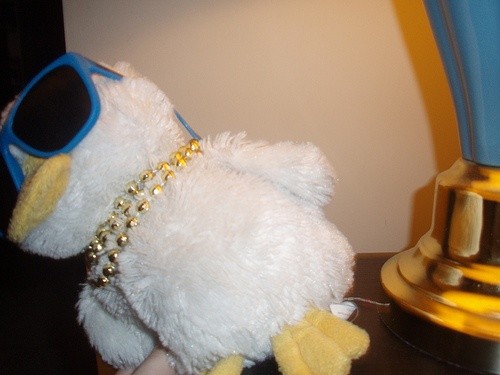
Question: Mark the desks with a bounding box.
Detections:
[85,252,479,375]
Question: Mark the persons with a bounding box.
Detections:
[116,346,178,375]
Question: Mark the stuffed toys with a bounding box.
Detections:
[1,51,370,375]
